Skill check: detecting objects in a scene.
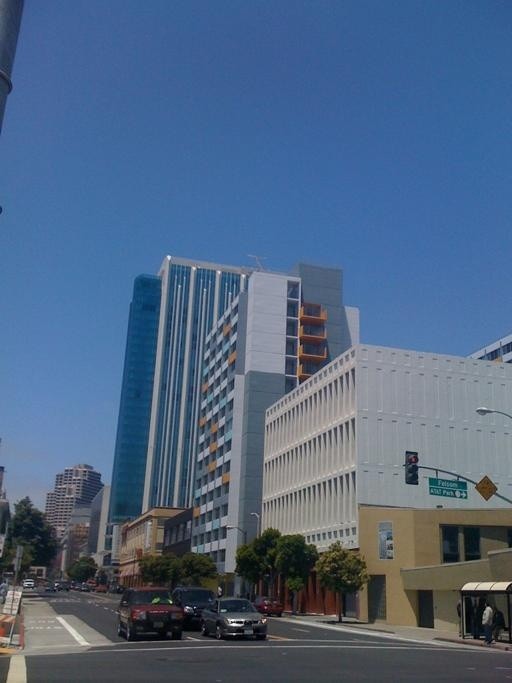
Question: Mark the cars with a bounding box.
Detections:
[109,583,285,642]
[22,578,107,594]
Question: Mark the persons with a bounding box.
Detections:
[480,600,494,643]
[490,606,505,641]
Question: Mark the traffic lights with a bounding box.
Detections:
[405,451,419,485]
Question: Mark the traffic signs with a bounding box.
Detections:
[429,477,467,499]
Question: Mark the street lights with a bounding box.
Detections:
[227,525,247,544]
[476,406,512,419]
[251,513,260,538]
